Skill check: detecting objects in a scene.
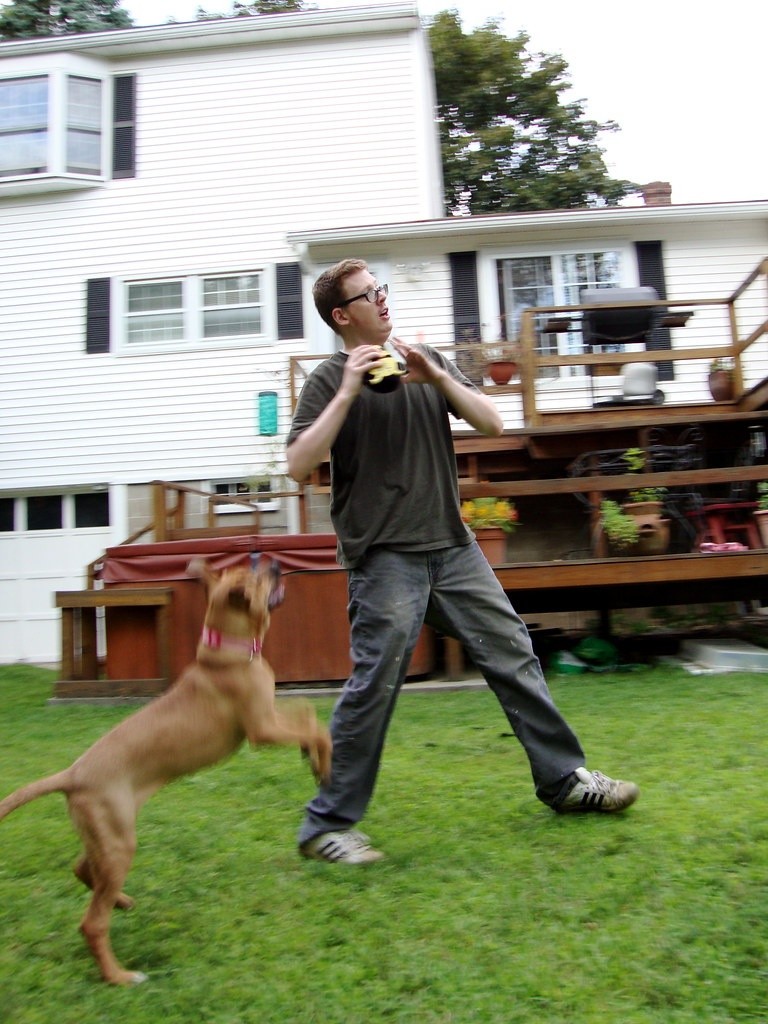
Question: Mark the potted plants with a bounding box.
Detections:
[752,482,768,548]
[617,445,670,555]
[455,312,542,386]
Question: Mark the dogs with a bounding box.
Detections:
[0,560,333,984]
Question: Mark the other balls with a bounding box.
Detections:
[363,348,407,393]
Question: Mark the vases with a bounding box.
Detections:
[470,527,506,564]
[708,369,734,402]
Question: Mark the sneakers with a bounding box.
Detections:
[302,829,385,865]
[557,767,638,813]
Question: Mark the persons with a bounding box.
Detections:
[284,259,638,863]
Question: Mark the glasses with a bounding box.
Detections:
[338,283,388,309]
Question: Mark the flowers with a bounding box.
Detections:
[460,479,524,535]
[709,357,747,389]
